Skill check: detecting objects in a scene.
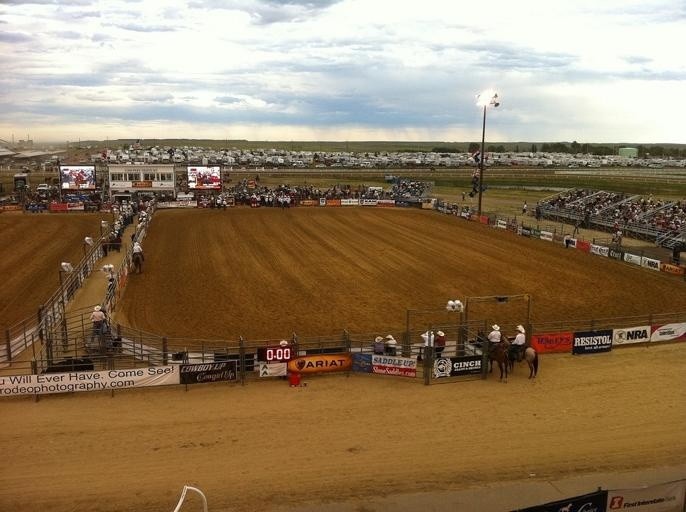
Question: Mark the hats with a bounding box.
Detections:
[375,337,383,342]
[94,305,101,311]
[516,325,525,333]
[492,325,500,331]
[437,331,444,336]
[386,335,393,339]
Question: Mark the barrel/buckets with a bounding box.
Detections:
[288,370,301,386]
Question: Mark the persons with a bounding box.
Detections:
[89,211,151,342]
[487,323,502,352]
[370,335,386,356]
[434,330,447,357]
[535,186,686,247]
[522,201,528,215]
[508,325,527,362]
[385,335,398,356]
[29,175,432,211]
[420,329,435,368]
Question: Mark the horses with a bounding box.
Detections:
[500,332,539,378]
[477,329,509,383]
[133,252,143,272]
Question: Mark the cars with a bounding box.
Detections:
[91,144,686,170]
[18,154,62,171]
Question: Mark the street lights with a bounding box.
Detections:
[475,89,500,215]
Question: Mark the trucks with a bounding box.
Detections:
[13,172,29,191]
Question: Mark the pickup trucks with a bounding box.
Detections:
[37,183,50,193]
